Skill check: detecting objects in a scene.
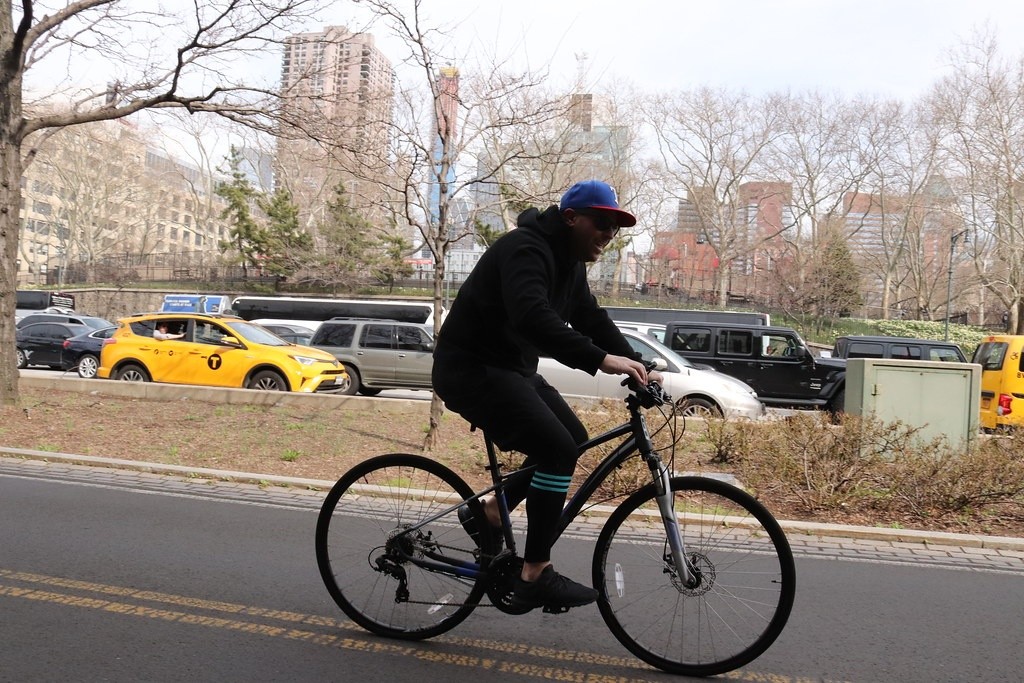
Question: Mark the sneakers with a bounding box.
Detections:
[512,565,601,612]
[458,499,506,561]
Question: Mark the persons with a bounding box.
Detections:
[154,324,187,341]
[432,181,665,607]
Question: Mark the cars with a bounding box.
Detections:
[250,319,318,346]
[536,329,766,421]
[14,323,96,368]
[60,325,119,378]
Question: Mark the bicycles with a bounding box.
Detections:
[314,370,795,677]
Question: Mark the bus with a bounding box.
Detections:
[16,288,76,328]
[601,304,772,360]
[231,294,444,337]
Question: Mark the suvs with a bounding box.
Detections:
[664,319,850,426]
[309,317,439,397]
[97,313,349,398]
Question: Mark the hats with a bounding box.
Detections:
[560,180,636,228]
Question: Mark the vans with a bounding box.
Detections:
[612,318,692,357]
[969,336,1024,434]
[830,335,973,363]
[17,311,114,334]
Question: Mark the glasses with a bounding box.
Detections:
[580,210,621,238]
